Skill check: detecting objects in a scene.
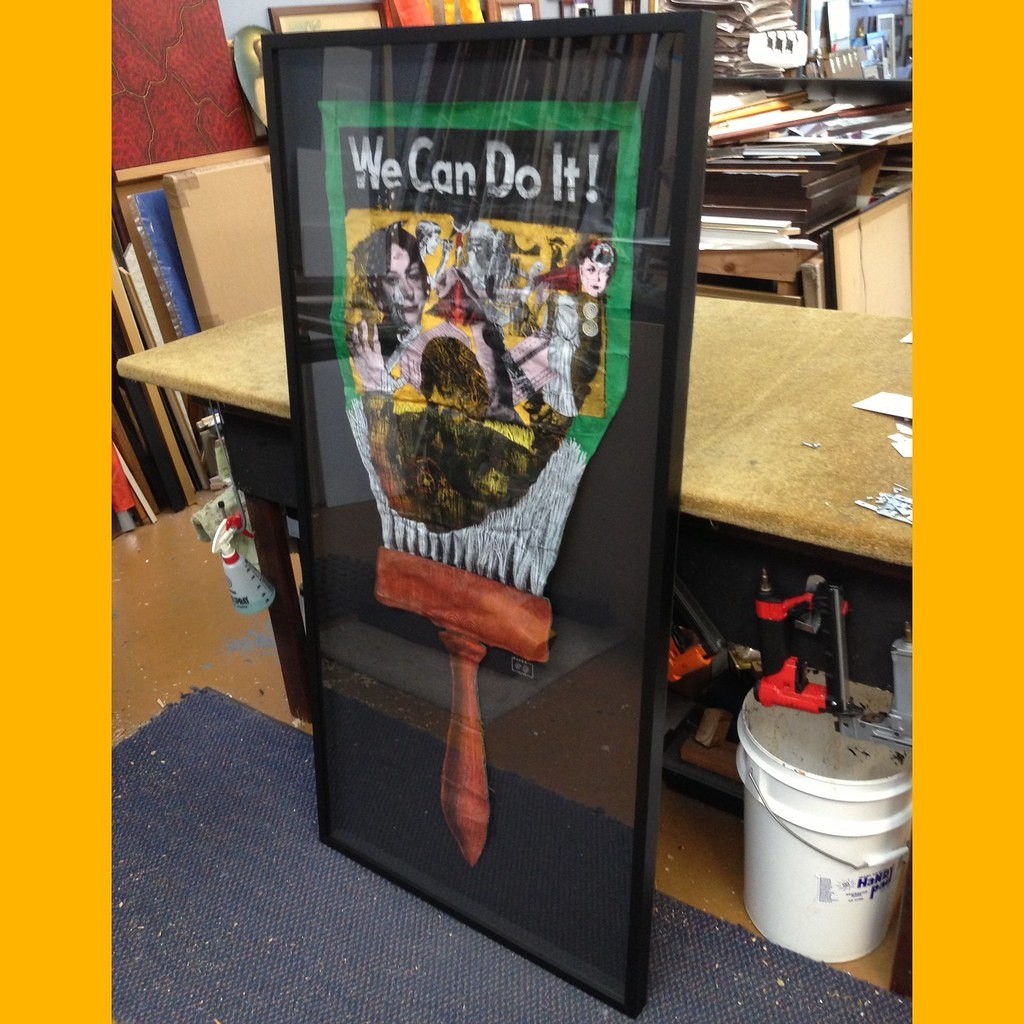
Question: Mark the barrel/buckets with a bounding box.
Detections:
[734,677,912,962]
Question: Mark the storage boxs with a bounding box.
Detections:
[162,154,283,331]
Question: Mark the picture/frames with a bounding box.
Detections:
[267,3,386,33]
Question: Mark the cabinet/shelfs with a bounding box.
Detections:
[632,1,913,169]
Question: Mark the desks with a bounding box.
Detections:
[109,293,913,720]
[704,141,888,244]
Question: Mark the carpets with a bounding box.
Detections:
[112,687,912,1024]
